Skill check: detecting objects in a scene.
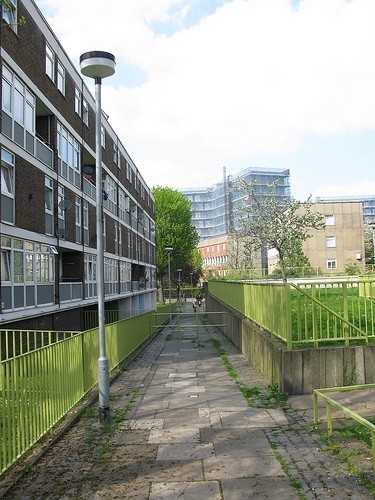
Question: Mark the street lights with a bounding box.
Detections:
[81,49,117,427]
[190,271,194,288]
[165,246,173,324]
[177,268,182,311]
